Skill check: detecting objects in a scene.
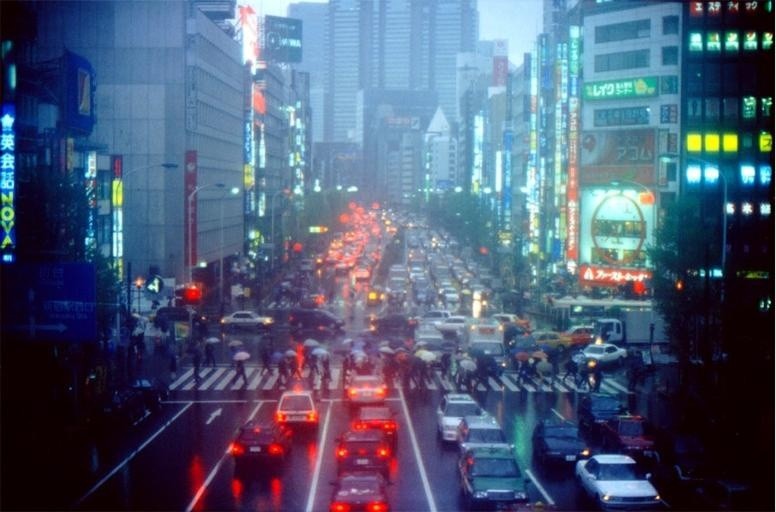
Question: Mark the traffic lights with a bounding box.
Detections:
[175,284,203,301]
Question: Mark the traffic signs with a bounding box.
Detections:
[144,274,164,294]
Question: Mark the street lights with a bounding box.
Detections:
[113,160,178,287]
[659,151,728,304]
[187,182,225,284]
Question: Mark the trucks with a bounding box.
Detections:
[596,309,671,353]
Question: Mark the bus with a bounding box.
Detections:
[543,299,605,330]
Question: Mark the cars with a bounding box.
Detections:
[593,414,664,462]
[458,447,531,509]
[353,405,398,449]
[578,393,630,433]
[532,331,573,354]
[568,325,597,341]
[582,343,628,369]
[457,415,505,444]
[575,452,662,509]
[274,389,319,429]
[335,429,393,482]
[533,419,590,464]
[436,392,484,442]
[347,375,388,405]
[232,418,294,473]
[220,310,274,331]
[328,470,395,511]
[290,207,534,367]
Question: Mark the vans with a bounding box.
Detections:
[154,308,209,335]
